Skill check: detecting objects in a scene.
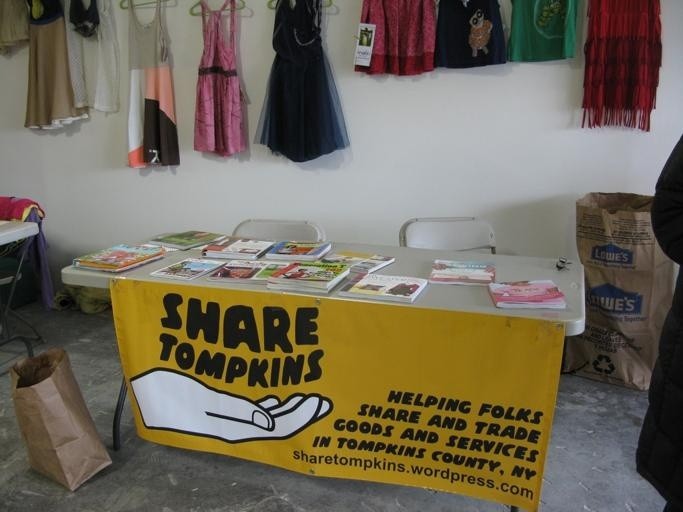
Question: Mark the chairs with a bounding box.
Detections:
[0,195,44,342]
[232,219,326,242]
[399,217,496,254]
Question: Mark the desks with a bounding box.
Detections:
[61,242,586,512]
[0,220,39,358]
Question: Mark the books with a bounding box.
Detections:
[489,279,568,309]
[427,259,495,286]
[72,229,428,304]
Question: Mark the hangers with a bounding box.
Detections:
[120,0,333,16]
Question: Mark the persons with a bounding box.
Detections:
[632,137,681,511]
